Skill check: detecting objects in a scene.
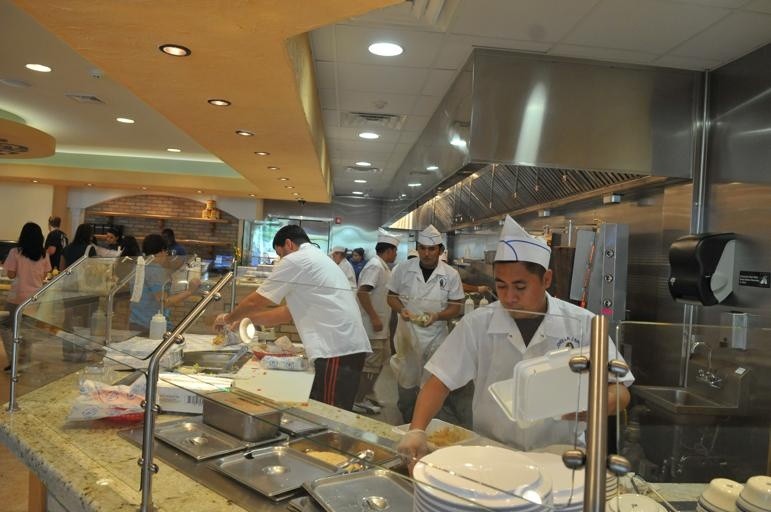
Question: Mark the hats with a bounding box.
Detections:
[494,215,551,270]
[377,227,403,247]
[328,246,346,254]
[419,224,442,247]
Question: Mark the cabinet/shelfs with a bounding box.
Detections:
[93,212,230,247]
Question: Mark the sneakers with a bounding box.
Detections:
[352,396,385,414]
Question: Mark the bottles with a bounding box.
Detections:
[478,296,489,310]
[52,266,59,279]
[465,295,475,316]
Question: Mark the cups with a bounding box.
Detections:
[243,275,256,282]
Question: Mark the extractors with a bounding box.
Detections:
[380,46,706,232]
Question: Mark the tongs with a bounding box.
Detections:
[338,448,375,473]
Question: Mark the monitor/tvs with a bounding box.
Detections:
[213,255,232,272]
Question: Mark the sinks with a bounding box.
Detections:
[630,384,734,418]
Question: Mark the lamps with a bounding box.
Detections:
[239,316,257,344]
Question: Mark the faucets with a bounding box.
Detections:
[689,340,713,371]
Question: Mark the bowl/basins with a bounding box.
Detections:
[695,474,771,512]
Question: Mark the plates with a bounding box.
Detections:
[240,279,258,283]
[605,491,666,512]
[531,444,620,512]
[252,347,305,362]
[411,442,553,512]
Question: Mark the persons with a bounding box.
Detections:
[332,232,400,414]
[395,214,636,478]
[45,216,68,271]
[213,225,374,410]
[386,223,464,423]
[60,224,204,336]
[0,222,52,373]
